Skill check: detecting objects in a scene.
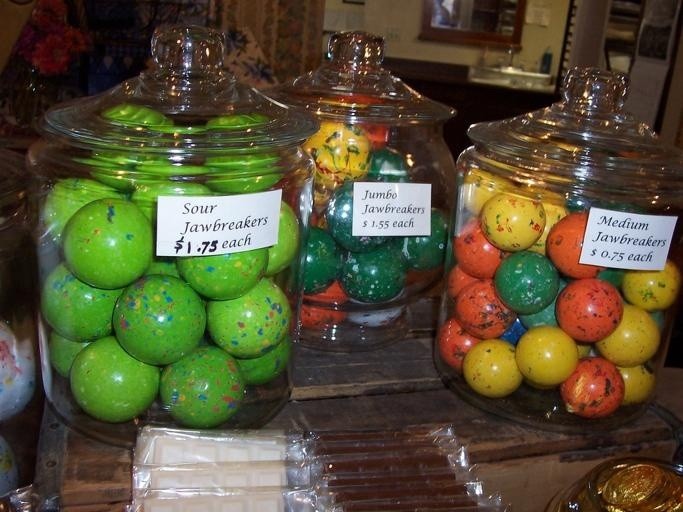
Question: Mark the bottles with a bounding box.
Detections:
[258,27,464,349]
[24,24,316,448]
[540,45,553,74]
[0,152,37,512]
[428,65,682,434]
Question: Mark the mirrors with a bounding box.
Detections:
[417,0,526,52]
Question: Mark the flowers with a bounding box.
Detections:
[15,13,103,78]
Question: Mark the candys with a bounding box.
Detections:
[441,142,683,419]
[287,92,446,327]
[0,94,299,429]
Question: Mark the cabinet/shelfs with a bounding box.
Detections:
[383,62,563,158]
